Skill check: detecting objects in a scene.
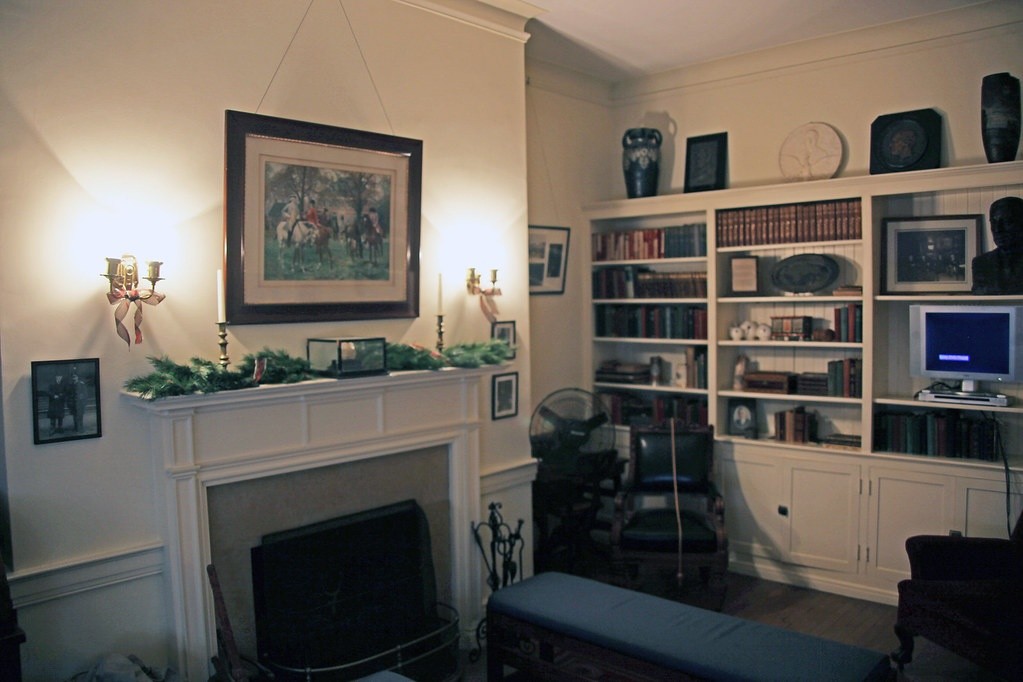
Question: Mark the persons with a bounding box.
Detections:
[46,371,88,436]
[971,197,1023,295]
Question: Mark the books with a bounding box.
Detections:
[775,405,862,448]
[873,410,1009,463]
[834,303,862,342]
[593,266,707,299]
[592,222,706,262]
[798,358,862,399]
[595,304,707,339]
[833,285,862,296]
[597,391,707,426]
[595,344,707,389]
[717,202,862,248]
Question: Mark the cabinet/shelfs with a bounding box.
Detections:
[578,160,1023,606]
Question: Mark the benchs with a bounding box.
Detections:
[487,572,896,682]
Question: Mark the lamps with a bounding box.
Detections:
[466,267,503,326]
[97,251,166,349]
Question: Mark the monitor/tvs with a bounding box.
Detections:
[909,304,1023,396]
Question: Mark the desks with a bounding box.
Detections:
[122,362,508,682]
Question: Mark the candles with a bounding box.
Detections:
[437,272,443,314]
[217,270,225,324]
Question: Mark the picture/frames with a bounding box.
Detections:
[221,107,422,325]
[30,356,102,446]
[728,399,756,435]
[527,224,571,295]
[879,213,981,296]
[491,322,516,360]
[683,131,729,192]
[491,370,519,420]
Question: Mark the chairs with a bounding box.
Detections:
[889,513,1023,682]
[609,419,730,612]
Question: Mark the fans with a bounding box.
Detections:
[529,386,617,482]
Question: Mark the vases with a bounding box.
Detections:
[622,127,663,199]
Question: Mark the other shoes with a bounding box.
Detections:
[48,427,64,437]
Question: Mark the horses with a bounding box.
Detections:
[361,216,383,264]
[276,220,333,274]
